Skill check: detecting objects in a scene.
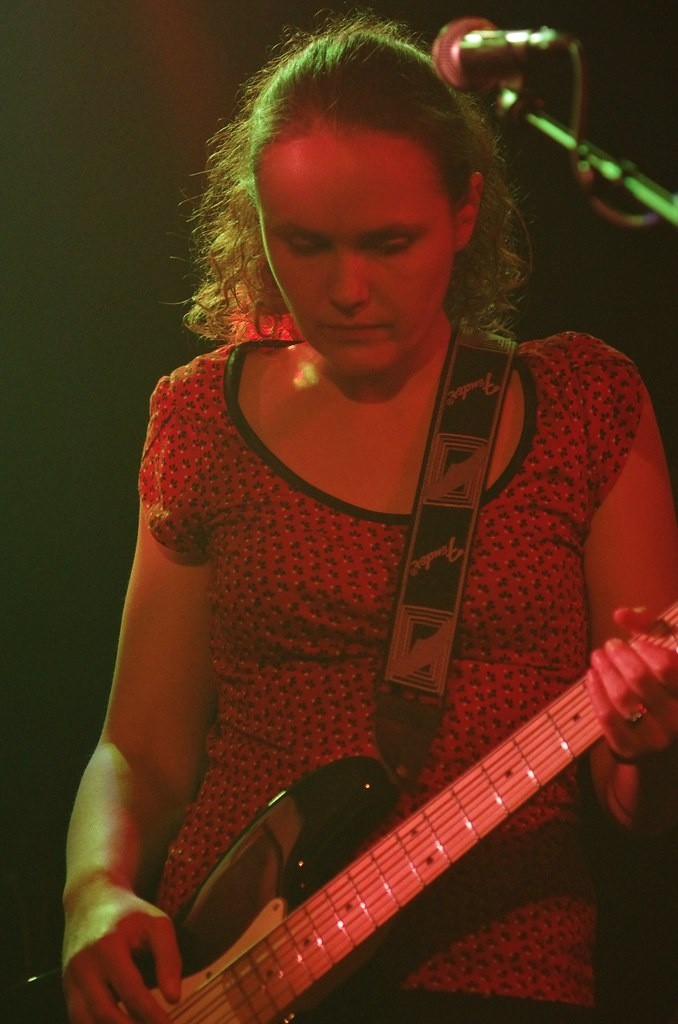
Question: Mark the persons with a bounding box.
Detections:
[61,27,678,1023]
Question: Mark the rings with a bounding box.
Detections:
[627,705,647,725]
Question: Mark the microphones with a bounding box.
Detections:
[432,17,576,92]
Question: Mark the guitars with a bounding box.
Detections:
[2,593,678,1024]
